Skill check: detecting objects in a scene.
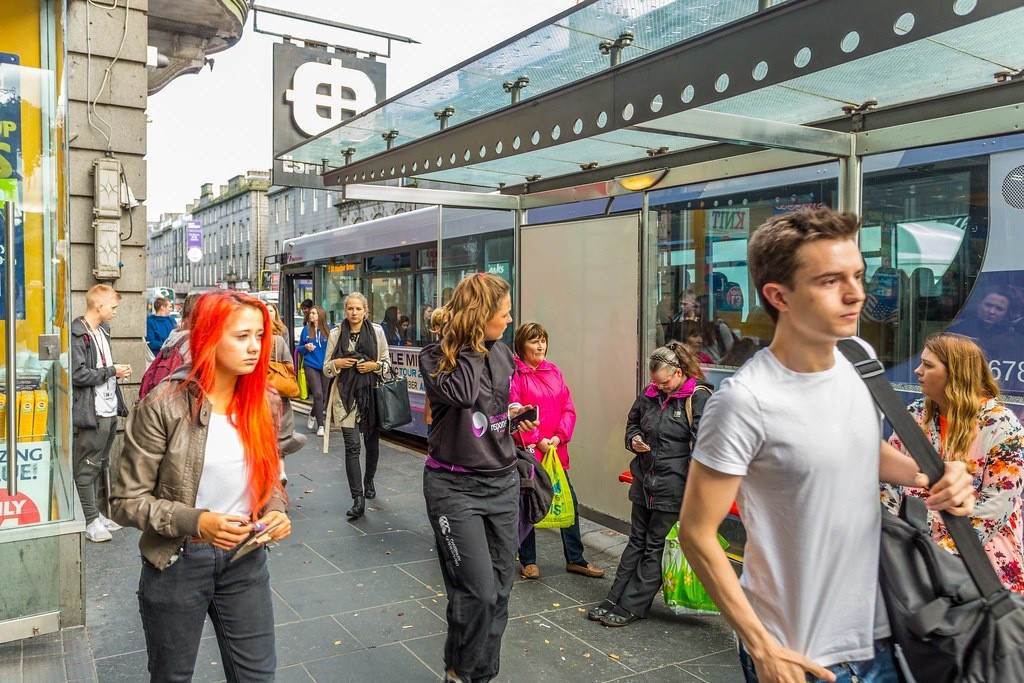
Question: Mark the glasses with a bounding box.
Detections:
[649,370,677,388]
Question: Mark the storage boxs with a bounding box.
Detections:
[0,389,50,443]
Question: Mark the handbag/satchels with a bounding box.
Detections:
[661,521,731,616]
[297,352,308,399]
[515,448,554,525]
[266,361,299,398]
[373,360,412,431]
[533,445,575,529]
[879,492,1024,683]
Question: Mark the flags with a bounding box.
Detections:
[861,289,898,324]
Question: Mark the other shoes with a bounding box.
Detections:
[566,561,605,577]
[280,473,288,489]
[600,605,638,627]
[521,563,539,579]
[588,598,618,620]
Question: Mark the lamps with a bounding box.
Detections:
[614,167,669,190]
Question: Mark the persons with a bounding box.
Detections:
[145,297,177,357]
[419,274,540,683]
[588,341,715,626]
[380,306,411,346]
[949,288,1011,340]
[675,320,714,363]
[266,302,294,486]
[510,323,605,578]
[159,291,202,363]
[108,288,291,683]
[677,207,977,683]
[656,272,734,358]
[323,292,390,521]
[71,284,123,543]
[421,304,433,347]
[882,333,1024,594]
[298,299,334,437]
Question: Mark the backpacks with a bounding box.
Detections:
[140,333,190,399]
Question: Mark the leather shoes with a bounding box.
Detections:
[347,496,364,517]
[364,477,376,499]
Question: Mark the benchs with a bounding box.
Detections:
[620,470,742,522]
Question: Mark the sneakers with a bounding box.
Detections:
[99,512,123,532]
[317,426,324,436]
[83,518,112,542]
[307,412,316,430]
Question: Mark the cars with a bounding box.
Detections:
[293,316,308,345]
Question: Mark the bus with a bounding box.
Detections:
[246,291,279,309]
[147,286,176,315]
[261,133,1024,543]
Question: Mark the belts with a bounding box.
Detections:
[182,534,208,543]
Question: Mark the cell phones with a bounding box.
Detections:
[632,438,648,447]
[357,358,365,363]
[510,405,539,434]
